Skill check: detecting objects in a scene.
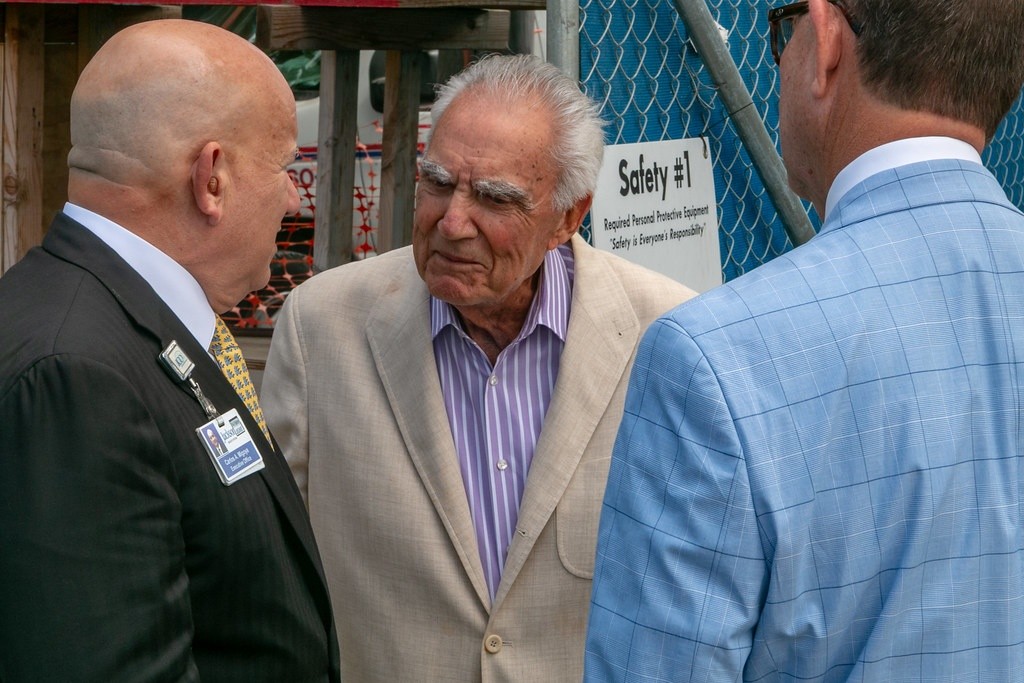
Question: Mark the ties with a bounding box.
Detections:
[206,312,275,456]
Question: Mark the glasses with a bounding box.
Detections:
[769,1,861,66]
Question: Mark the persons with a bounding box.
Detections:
[583,0,1024,683]
[0,17,341,683]
[259,57,700,683]
[206,428,228,457]
